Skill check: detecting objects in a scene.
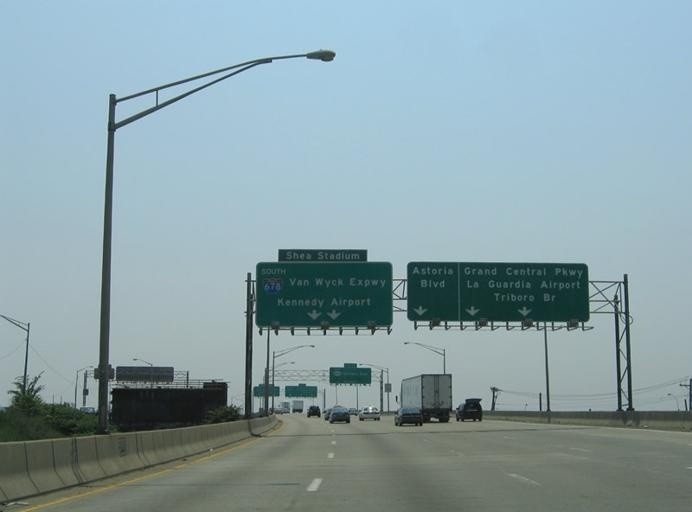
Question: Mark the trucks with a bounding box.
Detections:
[273,400,304,414]
[395,373,452,422]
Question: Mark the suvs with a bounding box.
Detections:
[455,399,482,421]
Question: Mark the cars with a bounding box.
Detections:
[306,405,381,424]
[394,407,423,427]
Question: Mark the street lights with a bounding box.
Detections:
[74,365,94,408]
[359,363,390,413]
[264,343,316,416]
[133,358,154,388]
[404,341,446,374]
